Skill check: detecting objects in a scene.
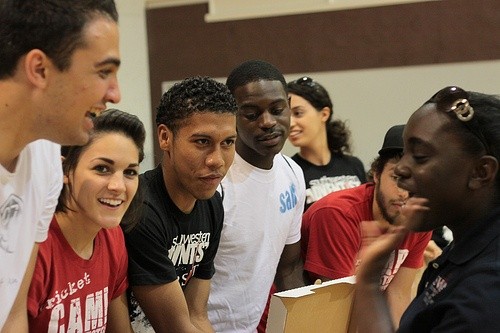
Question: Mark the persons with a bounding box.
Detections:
[25,108,146,333]
[0,0,121,333]
[120,76,237,333]
[206,61,500,333]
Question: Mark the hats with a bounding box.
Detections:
[378,124,405,154]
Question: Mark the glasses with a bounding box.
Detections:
[288,76,325,98]
[431,86,494,155]
